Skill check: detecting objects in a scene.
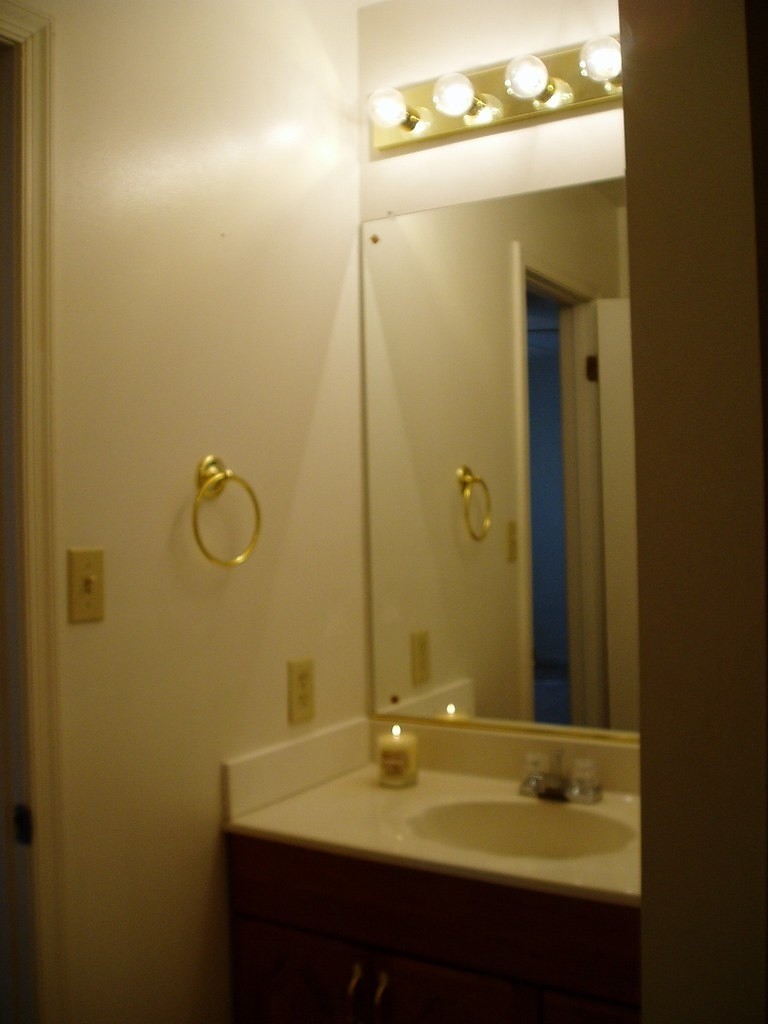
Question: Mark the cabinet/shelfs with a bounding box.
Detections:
[226,834,642,1024]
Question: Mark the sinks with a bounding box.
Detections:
[404,800,639,860]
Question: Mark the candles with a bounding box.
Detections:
[376,724,419,788]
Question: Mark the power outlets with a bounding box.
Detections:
[288,658,316,725]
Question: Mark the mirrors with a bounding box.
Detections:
[362,175,640,743]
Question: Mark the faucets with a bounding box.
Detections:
[515,772,573,803]
